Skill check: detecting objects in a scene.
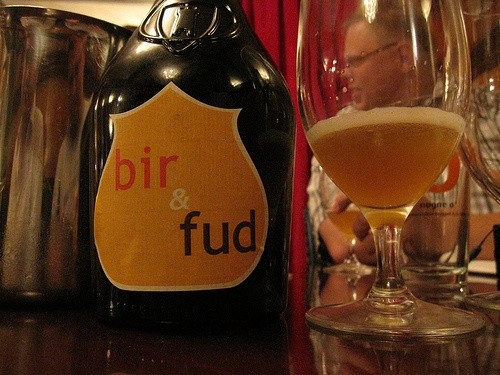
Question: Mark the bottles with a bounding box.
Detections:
[88,0,298,331]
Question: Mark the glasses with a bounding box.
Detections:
[344,41,401,69]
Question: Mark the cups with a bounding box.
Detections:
[1,5,134,315]
[441,0,500,204]
[389,94,470,306]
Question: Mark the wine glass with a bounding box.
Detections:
[292,0,487,341]
[319,166,380,276]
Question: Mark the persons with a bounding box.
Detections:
[307,1,500,271]
